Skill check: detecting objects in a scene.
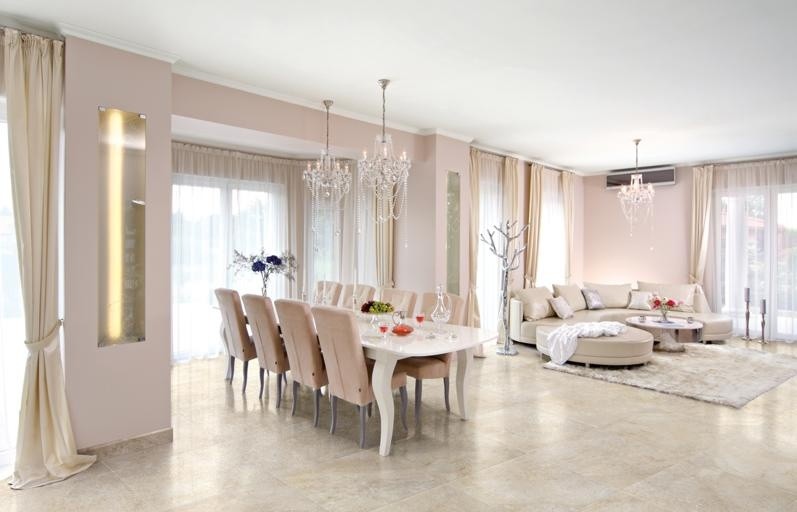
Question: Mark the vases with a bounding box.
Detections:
[360,300,395,314]
[368,314,392,329]
[659,311,669,323]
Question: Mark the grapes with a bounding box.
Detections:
[360,300,393,313]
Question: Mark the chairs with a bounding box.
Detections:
[313,281,343,305]
[240,294,324,408]
[380,289,417,316]
[399,292,468,412]
[215,289,288,391]
[307,304,422,446]
[275,299,367,424]
[341,285,379,312]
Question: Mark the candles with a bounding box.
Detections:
[745,288,750,301]
[761,299,766,313]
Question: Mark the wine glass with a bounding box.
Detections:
[378,321,389,343]
[416,312,425,330]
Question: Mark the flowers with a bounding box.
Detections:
[226,249,296,296]
[647,295,685,312]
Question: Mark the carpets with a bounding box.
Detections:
[544,344,796,410]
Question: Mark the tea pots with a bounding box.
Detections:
[430,284,452,330]
[391,310,414,337]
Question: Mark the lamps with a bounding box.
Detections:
[617,140,655,248]
[359,80,412,245]
[303,95,352,252]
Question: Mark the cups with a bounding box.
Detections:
[639,316,646,323]
[688,317,693,324]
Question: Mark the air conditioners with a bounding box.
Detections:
[606,166,678,190]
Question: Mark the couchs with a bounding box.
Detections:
[512,283,737,367]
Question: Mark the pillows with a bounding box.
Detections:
[512,281,696,322]
